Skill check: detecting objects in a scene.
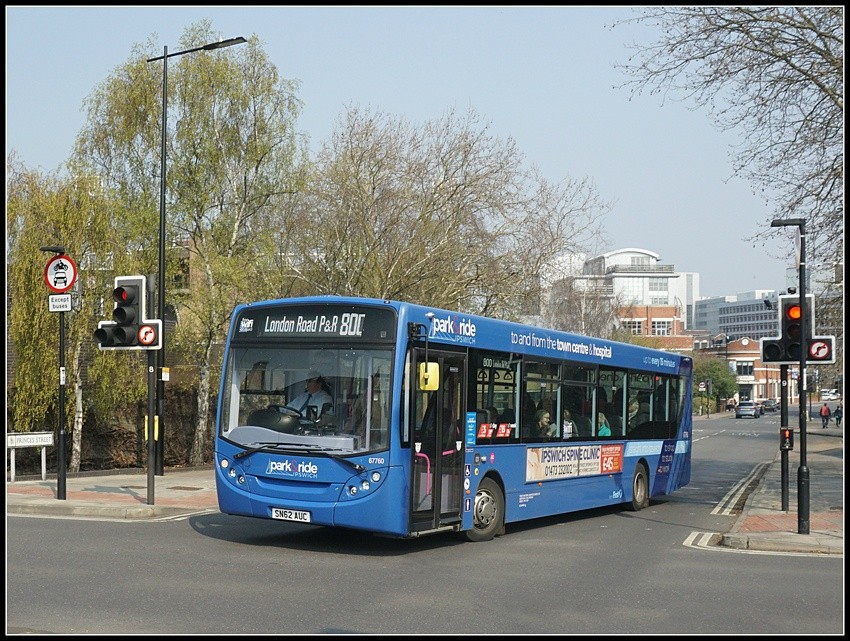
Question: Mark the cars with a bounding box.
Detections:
[762,399,781,412]
[755,401,765,415]
[735,401,760,418]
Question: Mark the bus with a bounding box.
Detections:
[214,293,695,543]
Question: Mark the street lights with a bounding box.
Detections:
[145,35,247,475]
[39,246,67,502]
[770,213,812,534]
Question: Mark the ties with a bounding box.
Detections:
[298,393,313,412]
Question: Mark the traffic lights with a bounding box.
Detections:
[760,294,815,363]
[777,425,794,452]
[92,275,147,351]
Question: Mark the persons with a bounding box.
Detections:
[627,397,649,436]
[530,409,550,437]
[834,406,842,427]
[820,403,831,429]
[656,375,677,401]
[564,410,579,437]
[346,394,362,417]
[524,392,536,421]
[598,413,611,436]
[280,370,333,425]
